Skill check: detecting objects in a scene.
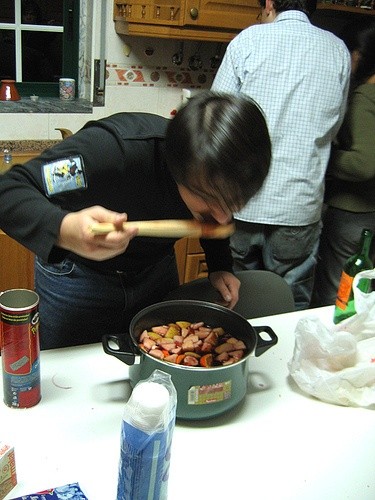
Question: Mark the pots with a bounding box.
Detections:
[103,300,278,423]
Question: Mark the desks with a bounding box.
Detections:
[1,300,374,500]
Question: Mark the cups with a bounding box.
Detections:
[58,78,75,101]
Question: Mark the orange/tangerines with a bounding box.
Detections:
[200,353,213,367]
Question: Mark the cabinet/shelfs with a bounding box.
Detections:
[114,0,264,44]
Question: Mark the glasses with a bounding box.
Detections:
[256,6,265,24]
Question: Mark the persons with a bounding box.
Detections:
[210,0,349,311]
[0,92,273,350]
[310,25,374,308]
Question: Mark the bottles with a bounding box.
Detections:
[116,383,175,500]
[333,229,372,323]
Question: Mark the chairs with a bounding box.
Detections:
[169,270,294,319]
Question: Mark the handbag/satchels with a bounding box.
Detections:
[289,270,375,406]
